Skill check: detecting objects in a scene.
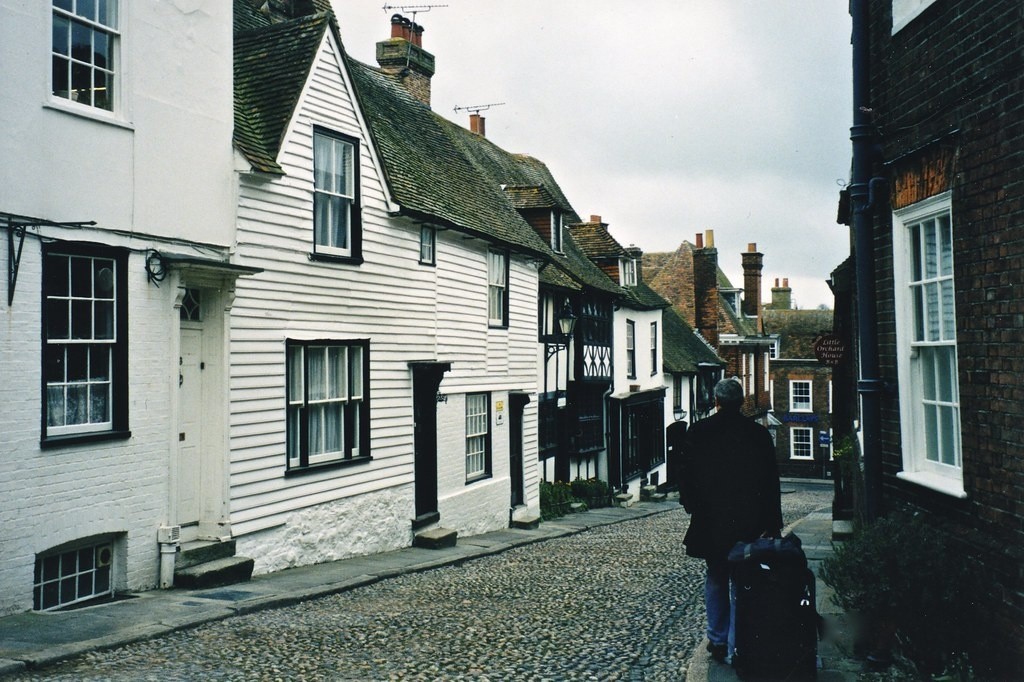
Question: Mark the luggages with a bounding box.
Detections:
[731,531,817,682]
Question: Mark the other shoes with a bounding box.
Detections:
[707,640,728,657]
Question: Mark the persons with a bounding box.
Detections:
[677,378,784,664]
